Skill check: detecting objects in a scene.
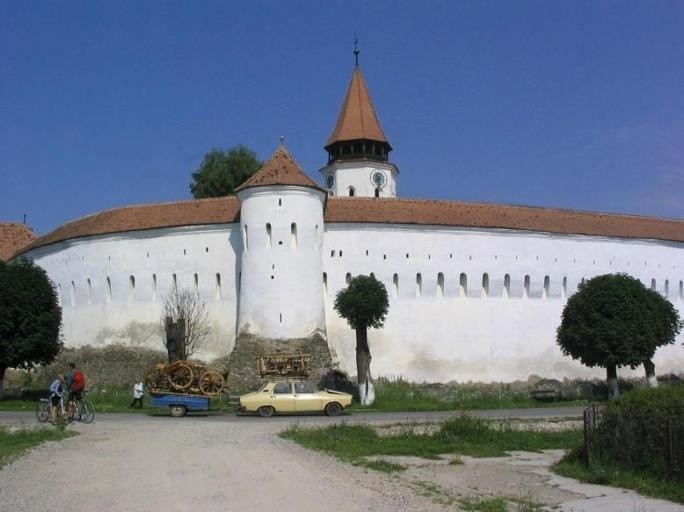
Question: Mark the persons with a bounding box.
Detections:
[61,361,85,421]
[49,372,66,428]
[128,378,145,409]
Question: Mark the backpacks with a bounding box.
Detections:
[71,370,85,392]
[57,383,63,393]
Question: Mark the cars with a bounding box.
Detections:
[240,375,352,417]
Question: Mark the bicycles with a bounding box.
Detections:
[35,391,72,425]
[147,361,225,397]
[61,388,95,423]
